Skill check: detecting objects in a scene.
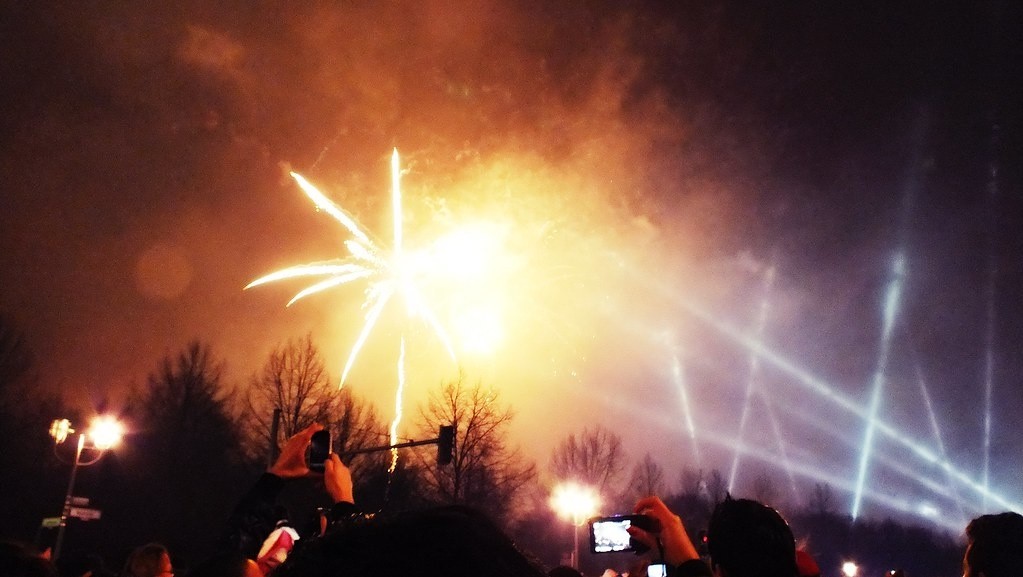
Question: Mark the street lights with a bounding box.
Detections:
[544,473,608,572]
[48,412,129,572]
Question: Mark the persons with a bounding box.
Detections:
[185,421,370,577]
[0,485,64,577]
[122,544,175,577]
[545,490,822,577]
[960,511,1023,577]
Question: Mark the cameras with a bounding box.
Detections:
[647,563,668,577]
[311,430,329,471]
[590,514,652,553]
